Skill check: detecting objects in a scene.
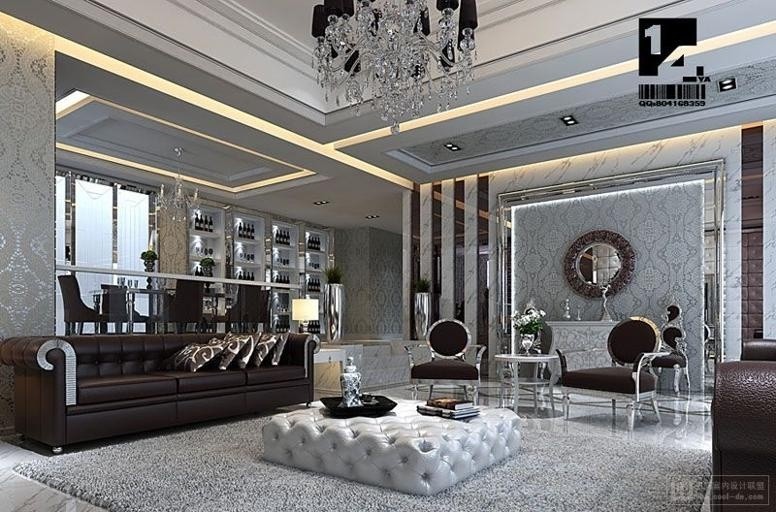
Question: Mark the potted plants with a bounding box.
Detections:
[319,264,345,345]
[140,249,158,271]
[200,257,214,278]
[411,278,433,340]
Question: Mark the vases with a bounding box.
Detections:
[519,333,537,358]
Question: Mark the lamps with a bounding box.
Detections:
[290,297,322,333]
[309,0,476,140]
[153,146,203,222]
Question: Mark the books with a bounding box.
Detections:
[415,398,481,420]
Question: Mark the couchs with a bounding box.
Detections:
[713,338,775,512]
[0,327,324,455]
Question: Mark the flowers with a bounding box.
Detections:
[510,308,547,336]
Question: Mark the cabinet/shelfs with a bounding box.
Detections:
[300,230,328,334]
[545,318,624,383]
[233,209,264,334]
[187,202,226,318]
[313,347,345,402]
[271,219,299,332]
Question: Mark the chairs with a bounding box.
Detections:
[403,317,487,406]
[149,279,204,332]
[101,284,145,332]
[647,302,692,394]
[210,284,261,334]
[56,274,98,336]
[261,288,272,331]
[544,314,663,425]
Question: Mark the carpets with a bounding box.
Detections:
[13,384,714,512]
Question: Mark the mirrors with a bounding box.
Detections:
[563,230,637,301]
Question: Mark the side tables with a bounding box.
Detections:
[494,352,558,417]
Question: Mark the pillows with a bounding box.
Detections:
[223,329,262,371]
[207,335,252,371]
[252,332,283,369]
[265,330,293,368]
[164,341,228,375]
